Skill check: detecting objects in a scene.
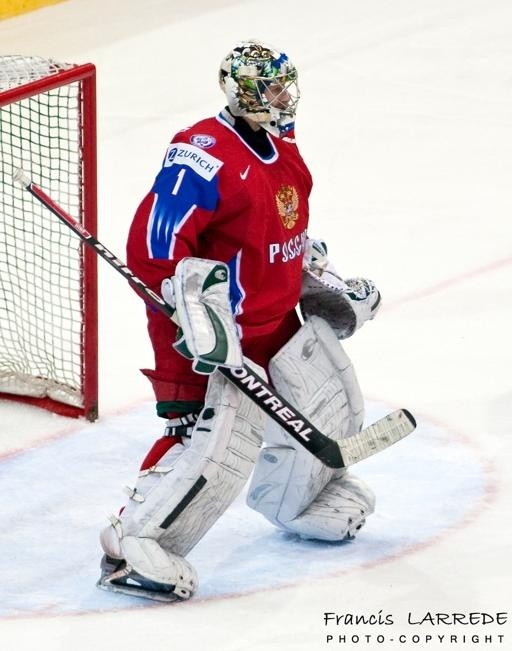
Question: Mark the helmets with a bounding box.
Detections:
[218,43,300,122]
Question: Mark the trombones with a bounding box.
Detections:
[10,169,419,467]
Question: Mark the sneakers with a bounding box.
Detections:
[97,523,196,596]
[276,476,375,542]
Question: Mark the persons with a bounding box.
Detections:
[101,41,378,592]
